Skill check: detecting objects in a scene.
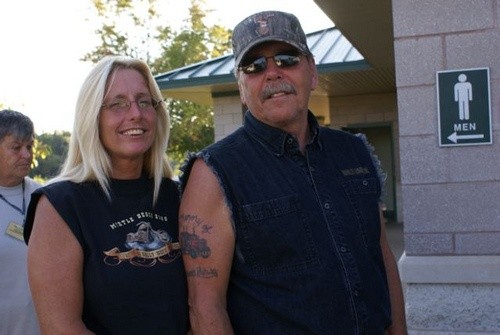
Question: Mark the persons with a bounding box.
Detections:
[23,55,192,335]
[179,12,407,335]
[0,109,47,335]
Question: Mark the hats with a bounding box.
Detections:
[232,11,308,70]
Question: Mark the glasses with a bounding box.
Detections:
[101,96,162,112]
[237,48,300,74]
